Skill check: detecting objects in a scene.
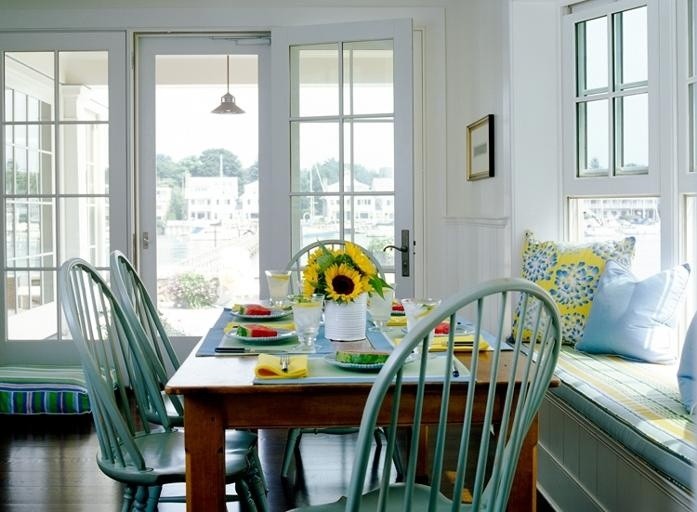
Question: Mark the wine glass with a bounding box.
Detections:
[286,294,324,352]
[369,283,397,333]
[400,297,441,360]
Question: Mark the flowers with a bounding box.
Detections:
[297,235,399,301]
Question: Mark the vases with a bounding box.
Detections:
[323,293,368,342]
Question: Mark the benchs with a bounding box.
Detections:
[507,343,696,511]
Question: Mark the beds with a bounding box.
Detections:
[1,363,119,441]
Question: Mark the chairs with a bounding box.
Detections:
[108,248,269,499]
[55,255,269,511]
[285,275,563,511]
[279,238,405,482]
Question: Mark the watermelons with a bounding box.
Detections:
[435,323,451,334]
[236,325,277,337]
[392,305,404,311]
[336,352,390,364]
[238,304,271,315]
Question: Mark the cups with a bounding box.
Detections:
[264,268,291,310]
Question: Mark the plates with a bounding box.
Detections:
[229,310,292,321]
[324,352,416,369]
[401,327,474,336]
[228,327,294,344]
[367,308,405,316]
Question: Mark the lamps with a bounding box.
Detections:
[210,55,246,115]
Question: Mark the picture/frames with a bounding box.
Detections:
[464,113,495,182]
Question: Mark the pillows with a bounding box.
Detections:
[503,226,637,347]
[573,257,696,370]
[675,311,696,418]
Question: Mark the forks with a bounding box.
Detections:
[279,351,290,372]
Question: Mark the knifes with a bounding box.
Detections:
[215,347,286,355]
[431,339,473,347]
